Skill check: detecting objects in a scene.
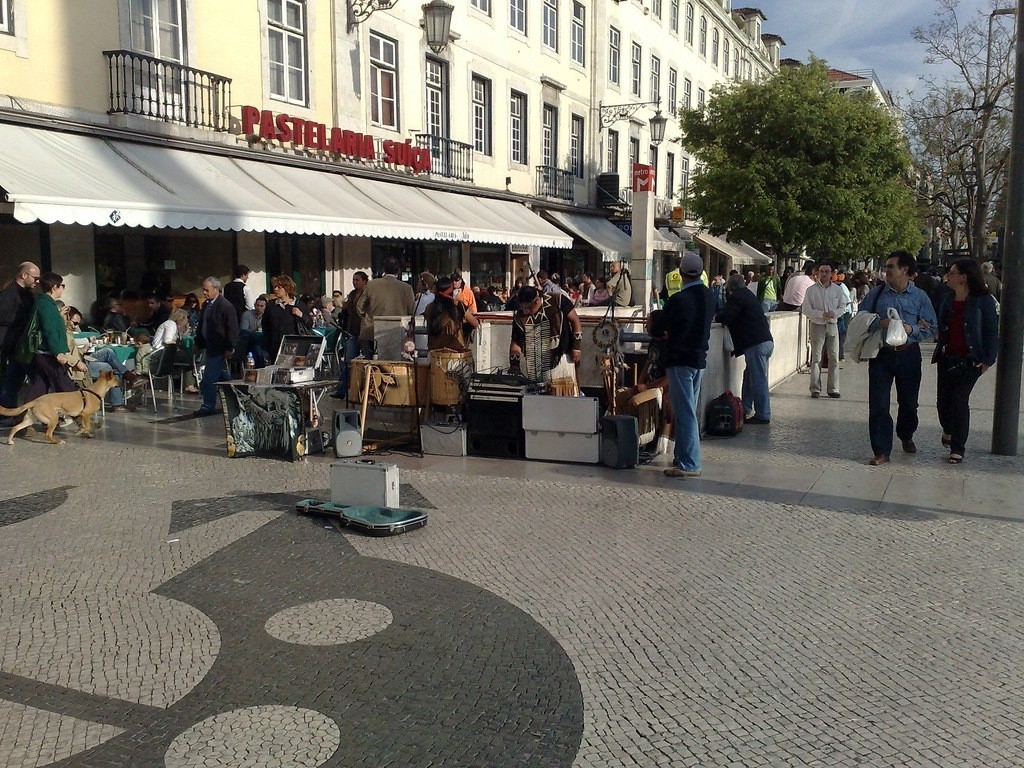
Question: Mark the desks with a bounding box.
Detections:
[94,342,138,406]
[212,378,339,459]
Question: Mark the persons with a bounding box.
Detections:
[92,272,241,417]
[659,256,709,301]
[222,264,313,368]
[831,267,886,369]
[710,261,816,312]
[918,256,999,463]
[651,253,717,477]
[715,273,774,424]
[0,261,149,440]
[859,250,938,466]
[299,271,374,400]
[914,259,1002,343]
[353,254,583,380]
[630,310,674,439]
[802,261,846,398]
[577,260,635,308]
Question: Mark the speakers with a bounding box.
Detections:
[332,409,363,458]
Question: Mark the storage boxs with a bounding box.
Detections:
[243,334,327,383]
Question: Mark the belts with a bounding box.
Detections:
[883,343,916,352]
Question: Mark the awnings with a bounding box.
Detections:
[540,208,657,262]
[0,121,573,250]
[653,226,687,252]
[681,226,773,270]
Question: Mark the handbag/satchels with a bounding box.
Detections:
[15,306,46,364]
[296,301,311,335]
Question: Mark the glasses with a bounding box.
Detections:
[26,273,39,281]
[948,271,960,276]
[884,265,902,269]
[71,319,78,325]
[58,283,66,289]
[453,279,461,282]
[820,270,831,274]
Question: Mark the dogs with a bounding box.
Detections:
[0,369,122,445]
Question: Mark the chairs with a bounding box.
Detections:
[73,326,337,418]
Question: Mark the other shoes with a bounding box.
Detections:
[129,377,149,388]
[812,392,818,398]
[193,410,214,417]
[664,469,701,477]
[828,393,840,398]
[870,454,890,465]
[900,437,916,452]
[744,416,770,424]
[110,405,136,413]
[330,391,345,399]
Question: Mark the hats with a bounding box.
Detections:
[681,251,702,276]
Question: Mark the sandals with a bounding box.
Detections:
[948,455,963,464]
[941,436,950,448]
